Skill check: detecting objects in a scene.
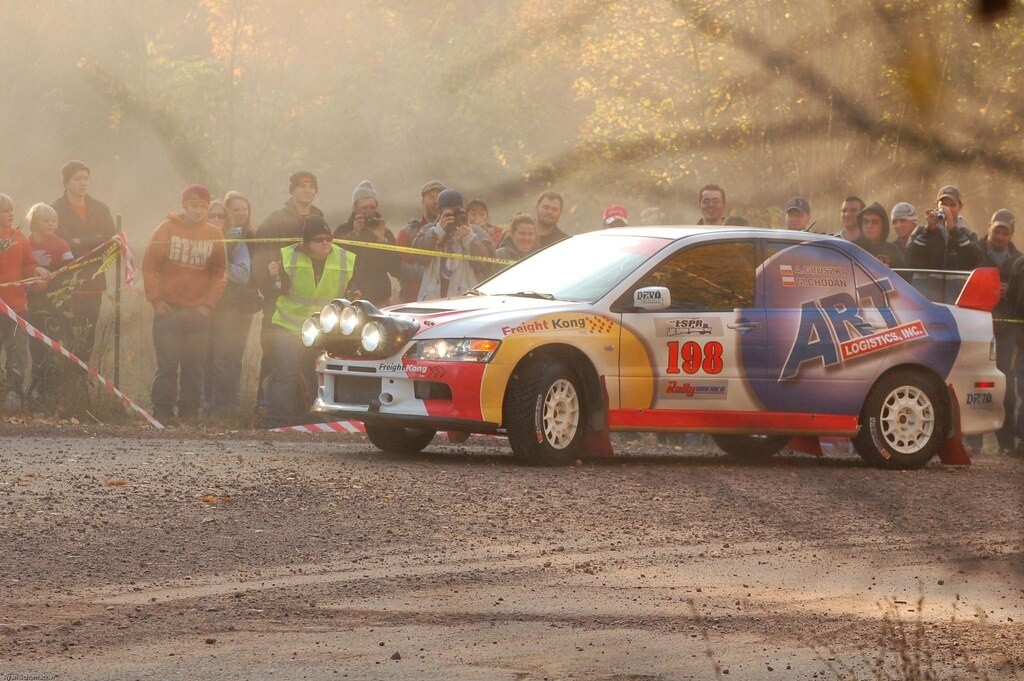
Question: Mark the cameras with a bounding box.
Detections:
[933,209,946,221]
[446,209,469,228]
[360,211,381,228]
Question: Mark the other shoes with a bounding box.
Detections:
[967,445,980,455]
[1002,446,1017,458]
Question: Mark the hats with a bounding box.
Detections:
[437,188,463,208]
[181,185,210,208]
[289,171,317,193]
[786,198,810,215]
[992,208,1015,232]
[302,215,331,241]
[61,160,89,188]
[421,180,447,193]
[938,185,962,206]
[892,202,918,223]
[352,181,379,208]
[602,205,628,225]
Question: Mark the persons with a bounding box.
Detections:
[0,159,117,414]
[138,171,629,425]
[695,184,1024,458]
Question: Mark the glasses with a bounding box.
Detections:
[208,213,226,221]
[310,236,333,244]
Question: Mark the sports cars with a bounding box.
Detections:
[297,224,1010,472]
